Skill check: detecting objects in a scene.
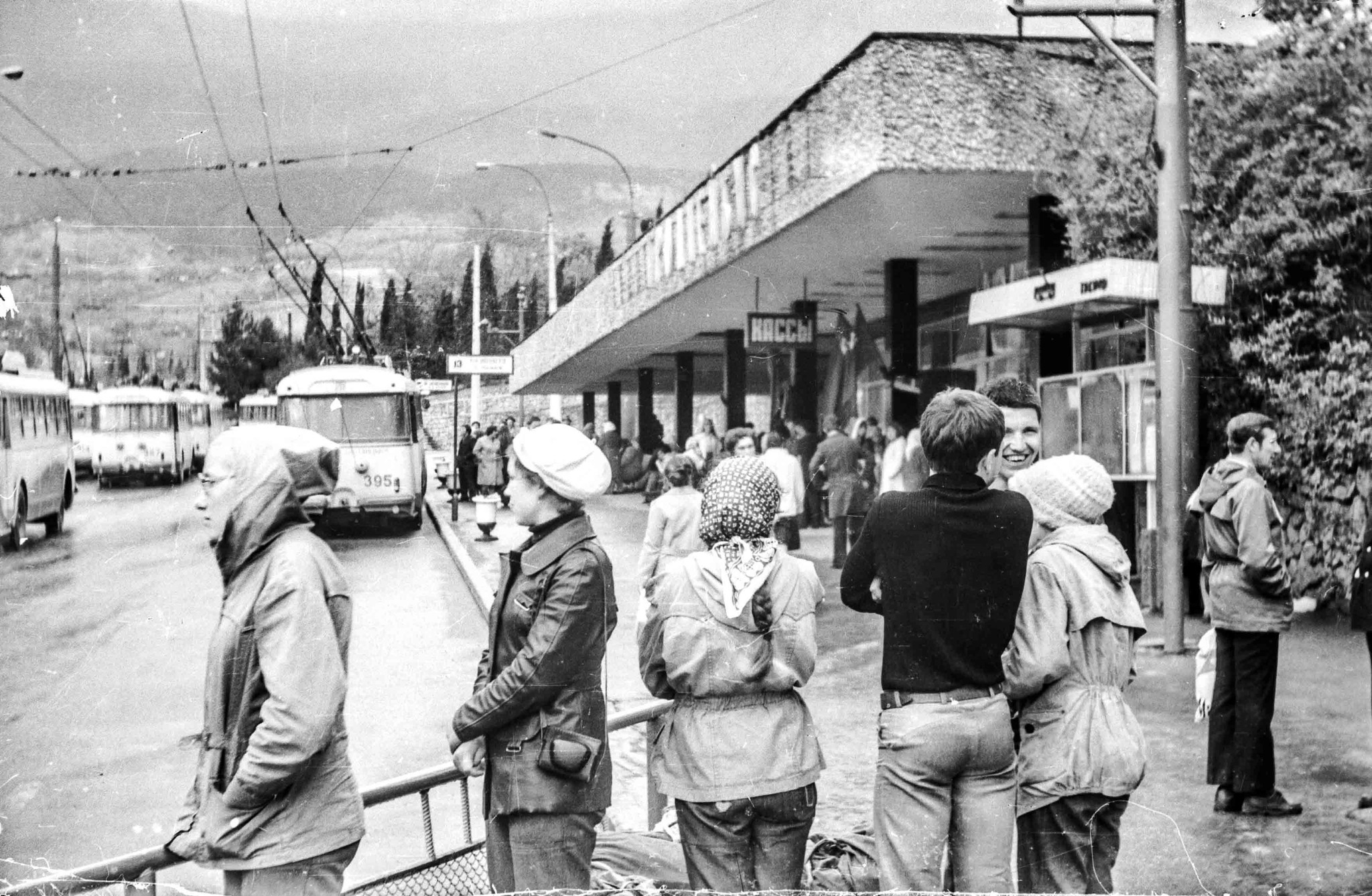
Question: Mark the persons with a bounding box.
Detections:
[1186,411,1304,817]
[725,427,756,457]
[637,456,824,896]
[501,417,516,484]
[471,422,480,441]
[1002,451,1149,895]
[684,418,731,473]
[583,413,677,504]
[765,432,805,551]
[472,425,504,497]
[849,416,931,508]
[809,415,873,569]
[167,426,364,896]
[1348,513,1371,809]
[447,424,618,895]
[529,416,540,429]
[798,418,829,529]
[840,389,1035,895]
[457,425,476,503]
[975,377,1041,492]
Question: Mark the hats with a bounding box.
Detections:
[1009,451,1114,528]
[513,423,612,502]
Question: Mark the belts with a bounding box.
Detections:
[879,685,1002,709]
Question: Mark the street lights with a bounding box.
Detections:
[476,162,558,314]
[540,129,634,248]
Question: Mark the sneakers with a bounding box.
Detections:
[1214,785,1242,811]
[1243,788,1301,815]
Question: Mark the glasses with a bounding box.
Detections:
[197,471,221,494]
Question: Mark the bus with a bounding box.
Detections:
[237,201,430,536]
[0,352,78,553]
[59,307,228,488]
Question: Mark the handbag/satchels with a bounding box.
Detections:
[1195,627,1216,724]
[538,727,603,782]
